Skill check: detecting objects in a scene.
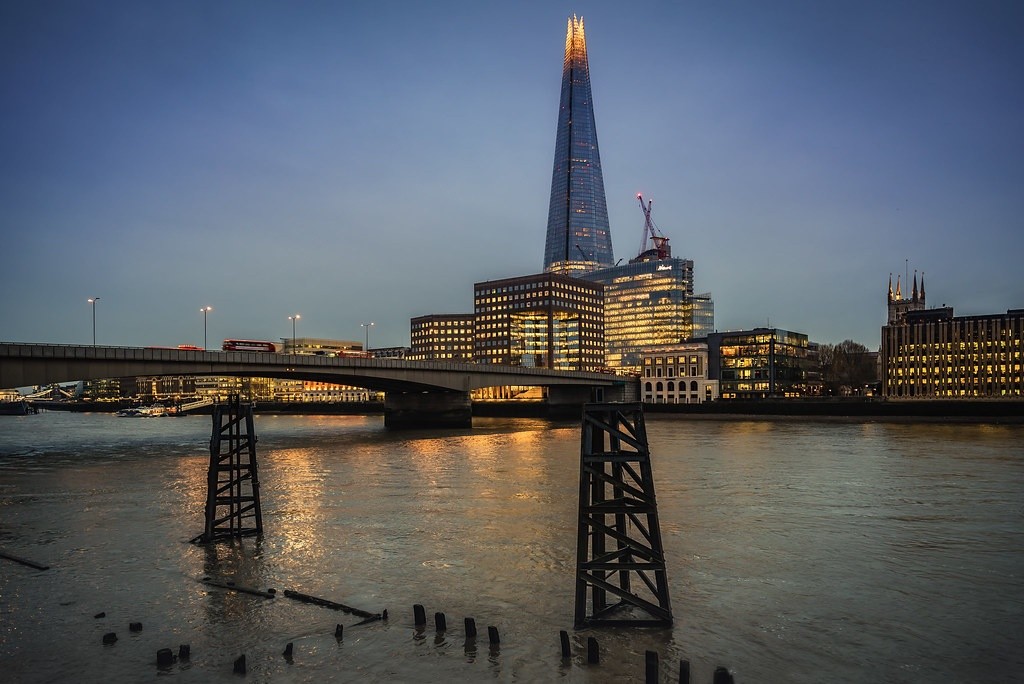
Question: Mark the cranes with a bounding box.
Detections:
[636,194,657,256]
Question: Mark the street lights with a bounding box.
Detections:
[361,322,374,351]
[88,297,101,347]
[288,314,300,355]
[200,307,212,350]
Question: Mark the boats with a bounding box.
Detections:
[115,401,169,417]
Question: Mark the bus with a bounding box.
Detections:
[223,339,276,353]
